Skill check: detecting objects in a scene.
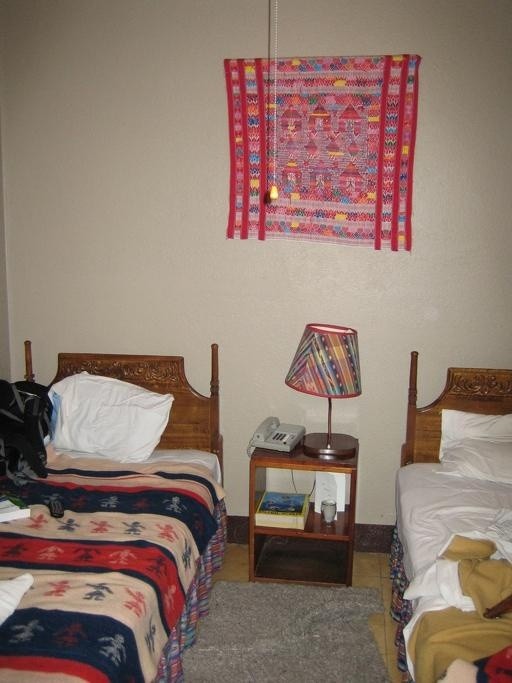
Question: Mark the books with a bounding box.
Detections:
[255,492,310,531]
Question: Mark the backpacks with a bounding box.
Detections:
[0,380,53,485]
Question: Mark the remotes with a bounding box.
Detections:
[51,495,64,517]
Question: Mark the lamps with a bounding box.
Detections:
[282,323,363,457]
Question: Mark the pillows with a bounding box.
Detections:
[43,370,176,464]
[437,406,512,482]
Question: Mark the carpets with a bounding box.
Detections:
[179,569,388,681]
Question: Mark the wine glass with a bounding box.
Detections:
[321,499,337,527]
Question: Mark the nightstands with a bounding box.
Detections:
[248,446,353,588]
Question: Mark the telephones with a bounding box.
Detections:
[250,417,306,452]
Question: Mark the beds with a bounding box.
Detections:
[396,350,512,682]
[1,340,226,683]
